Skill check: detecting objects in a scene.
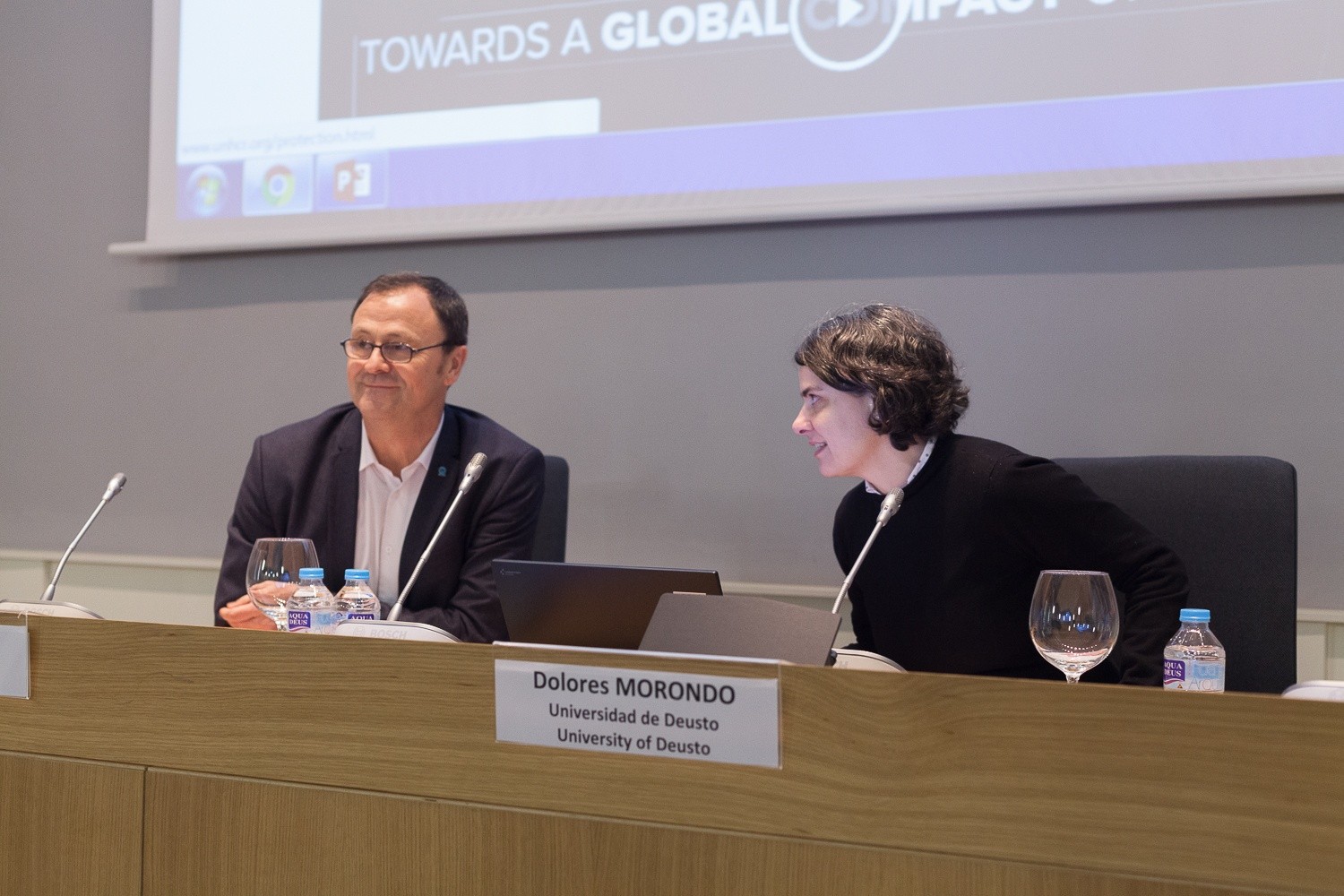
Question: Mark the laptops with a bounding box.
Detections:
[489,560,723,651]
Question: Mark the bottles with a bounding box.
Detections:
[1163,610,1226,694]
[285,567,337,635]
[331,569,381,622]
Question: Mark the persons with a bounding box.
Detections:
[214,272,545,644]
[791,303,1188,691]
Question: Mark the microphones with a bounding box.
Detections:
[830,486,906,672]
[336,451,489,643]
[0,473,127,621]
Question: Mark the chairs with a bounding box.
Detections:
[1048,453,1297,696]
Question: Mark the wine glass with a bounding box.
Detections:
[245,538,321,631]
[1028,570,1120,685]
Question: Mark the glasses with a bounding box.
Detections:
[341,340,458,365]
